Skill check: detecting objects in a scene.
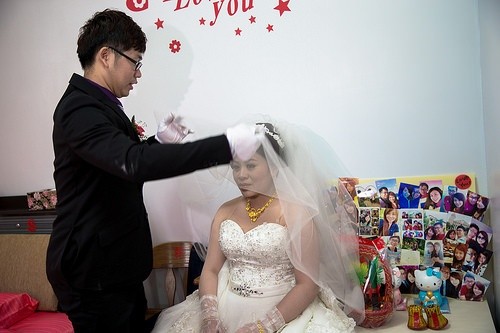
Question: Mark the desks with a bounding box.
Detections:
[355,294,498,333]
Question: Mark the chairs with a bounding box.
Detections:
[144,242,207,333]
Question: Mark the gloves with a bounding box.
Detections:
[227,123,264,162]
[154,113,197,147]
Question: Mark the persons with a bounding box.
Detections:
[153,114,365,332]
[345,180,490,301]
[45,8,266,333]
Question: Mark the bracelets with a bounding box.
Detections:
[255,319,266,333]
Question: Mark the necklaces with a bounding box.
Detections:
[245,193,277,222]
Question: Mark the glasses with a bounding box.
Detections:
[106,45,143,72]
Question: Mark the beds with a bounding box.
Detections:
[0,210,76,333]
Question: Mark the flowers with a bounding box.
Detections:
[131,115,148,142]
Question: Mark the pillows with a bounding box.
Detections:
[0,292,40,329]
